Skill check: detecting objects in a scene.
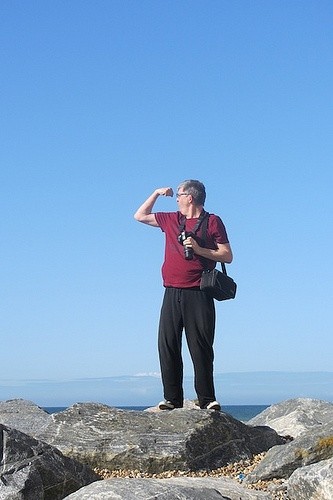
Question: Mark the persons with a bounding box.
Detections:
[133,179,233,409]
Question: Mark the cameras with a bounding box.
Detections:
[178,231,199,260]
